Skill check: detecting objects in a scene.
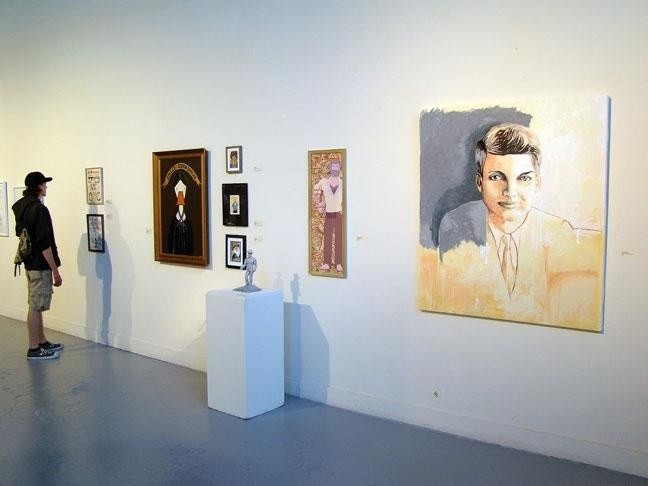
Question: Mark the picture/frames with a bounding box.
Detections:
[85,167,103,205]
[226,146,242,173]
[222,183,248,226]
[0,181,9,237]
[13,187,44,205]
[86,214,105,252]
[152,147,208,266]
[308,149,347,278]
[226,234,246,269]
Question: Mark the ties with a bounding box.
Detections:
[500,232,518,300]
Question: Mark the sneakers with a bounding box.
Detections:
[26,349,59,360]
[40,342,64,351]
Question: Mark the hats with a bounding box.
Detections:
[25,172,53,184]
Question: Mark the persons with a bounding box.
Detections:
[231,241,241,262]
[311,160,343,272]
[231,197,239,213]
[240,249,257,289]
[437,121,577,324]
[229,151,238,168]
[11,171,65,360]
[166,180,193,256]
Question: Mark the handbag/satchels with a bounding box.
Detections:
[13,227,31,278]
